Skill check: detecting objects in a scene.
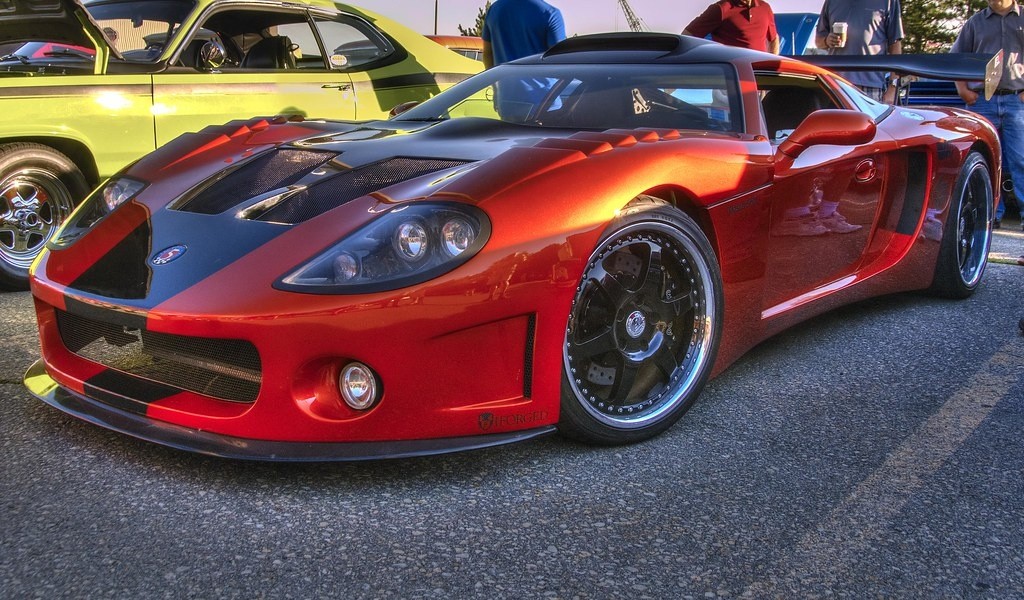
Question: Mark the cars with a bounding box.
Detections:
[334,35,486,76]
[0,1,500,292]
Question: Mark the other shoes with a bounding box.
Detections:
[1019,217,1024,231]
[993,215,1001,229]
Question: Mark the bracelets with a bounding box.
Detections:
[886,78,898,88]
[824,36,829,48]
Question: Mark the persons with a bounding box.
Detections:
[481,0,566,125]
[947,0,1024,233]
[663,0,781,107]
[814,0,905,105]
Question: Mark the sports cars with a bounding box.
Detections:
[22,29,1004,466]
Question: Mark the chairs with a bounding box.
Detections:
[241,36,295,69]
[763,86,823,139]
[570,81,635,127]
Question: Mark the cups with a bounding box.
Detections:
[834,22,848,48]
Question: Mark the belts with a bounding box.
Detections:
[980,88,1024,96]
